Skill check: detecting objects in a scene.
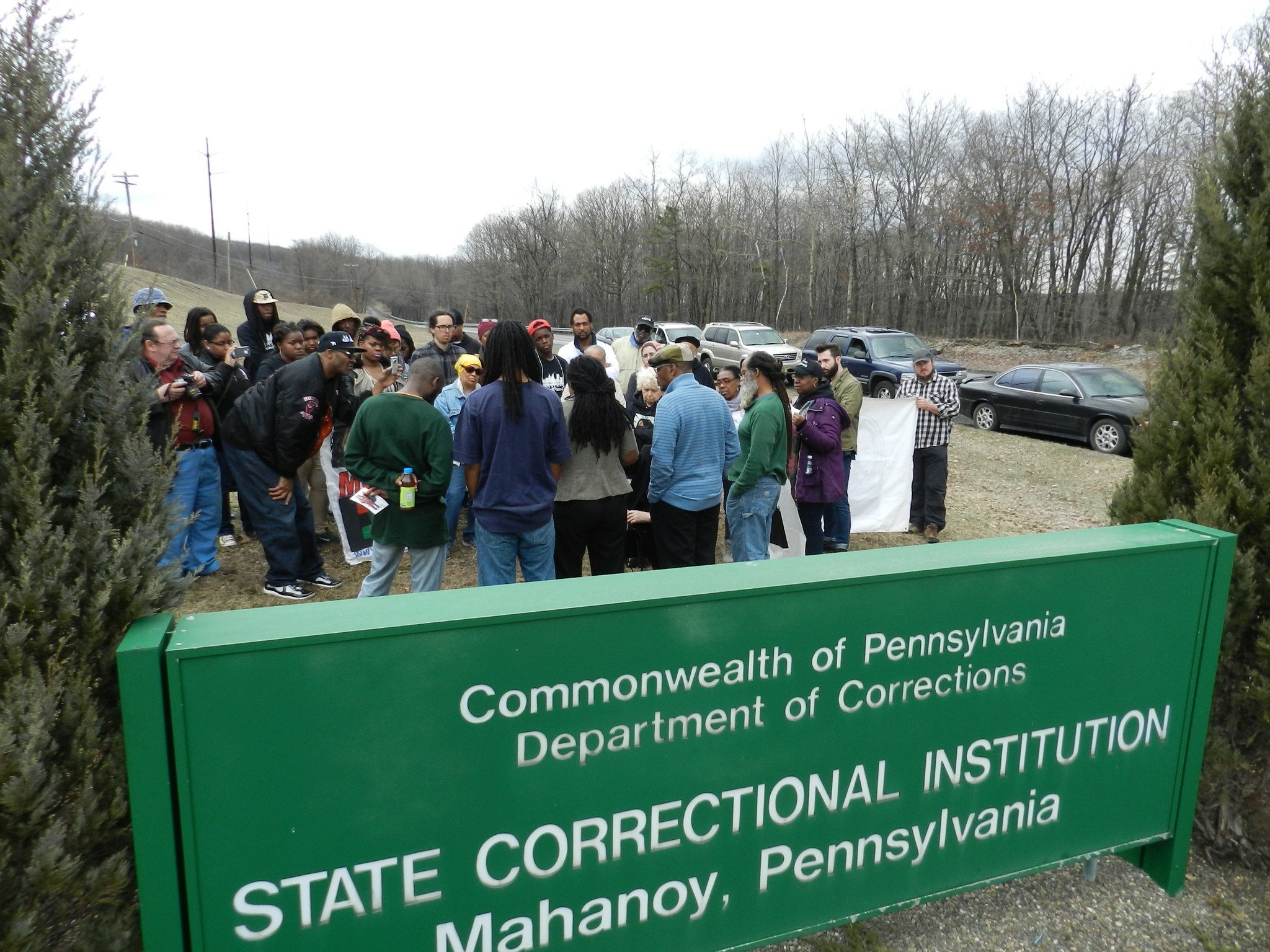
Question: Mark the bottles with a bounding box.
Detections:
[805,455,813,474]
[399,468,416,510]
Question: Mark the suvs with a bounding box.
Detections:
[802,325,967,399]
[699,320,802,387]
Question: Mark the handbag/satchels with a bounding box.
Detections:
[624,522,649,568]
[769,502,790,549]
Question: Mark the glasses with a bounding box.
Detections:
[460,364,483,376]
[636,325,653,333]
[479,318,500,323]
[791,374,813,380]
[641,352,657,359]
[435,325,457,331]
[714,378,737,386]
[211,339,235,346]
[151,337,182,347]
[384,345,402,353]
[654,364,671,373]
[739,371,746,378]
[336,349,357,359]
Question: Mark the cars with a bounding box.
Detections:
[957,362,1161,457]
[652,321,703,357]
[595,326,665,349]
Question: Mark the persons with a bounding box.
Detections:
[451,320,572,589]
[720,350,794,562]
[644,342,741,571]
[892,349,959,545]
[127,320,238,579]
[220,331,367,601]
[120,286,863,600]
[342,357,453,598]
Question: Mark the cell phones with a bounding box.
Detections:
[232,346,251,358]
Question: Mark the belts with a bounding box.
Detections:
[174,441,214,450]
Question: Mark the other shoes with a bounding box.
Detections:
[297,574,343,589]
[824,540,847,553]
[373,504,380,510]
[904,525,922,535]
[219,534,237,547]
[316,533,341,544]
[462,539,479,550]
[263,580,315,600]
[202,568,238,578]
[923,523,941,543]
[445,549,452,560]
[722,538,735,563]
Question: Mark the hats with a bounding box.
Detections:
[675,334,701,348]
[478,319,499,340]
[381,319,402,343]
[317,331,367,353]
[252,289,278,304]
[455,354,482,377]
[528,319,551,335]
[133,288,173,314]
[358,322,391,345]
[446,308,464,325]
[636,315,655,328]
[912,348,932,365]
[787,358,823,381]
[648,343,693,364]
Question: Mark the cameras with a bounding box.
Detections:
[390,356,399,375]
[173,373,202,402]
